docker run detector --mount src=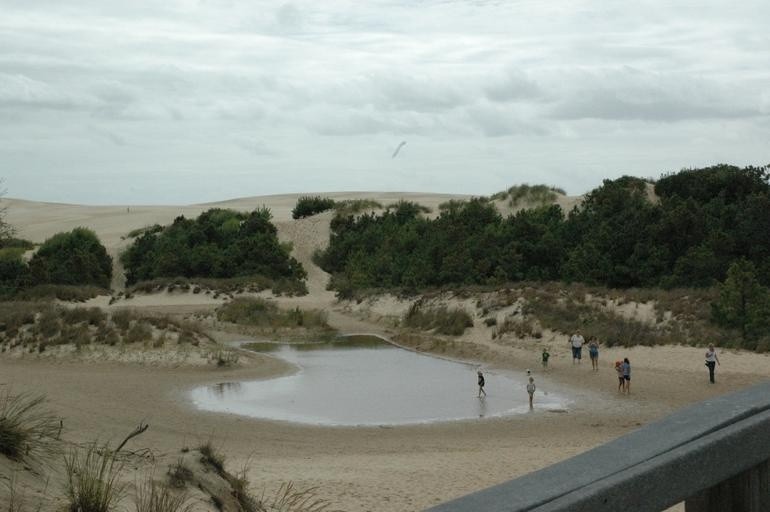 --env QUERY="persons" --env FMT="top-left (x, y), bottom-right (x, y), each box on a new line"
top-left (527, 377), bottom-right (536, 408)
top-left (477, 371), bottom-right (486, 398)
top-left (615, 358), bottom-right (631, 394)
top-left (571, 329), bottom-right (585, 365)
top-left (543, 348), bottom-right (550, 368)
top-left (587, 335), bottom-right (599, 370)
top-left (706, 343), bottom-right (720, 384)
